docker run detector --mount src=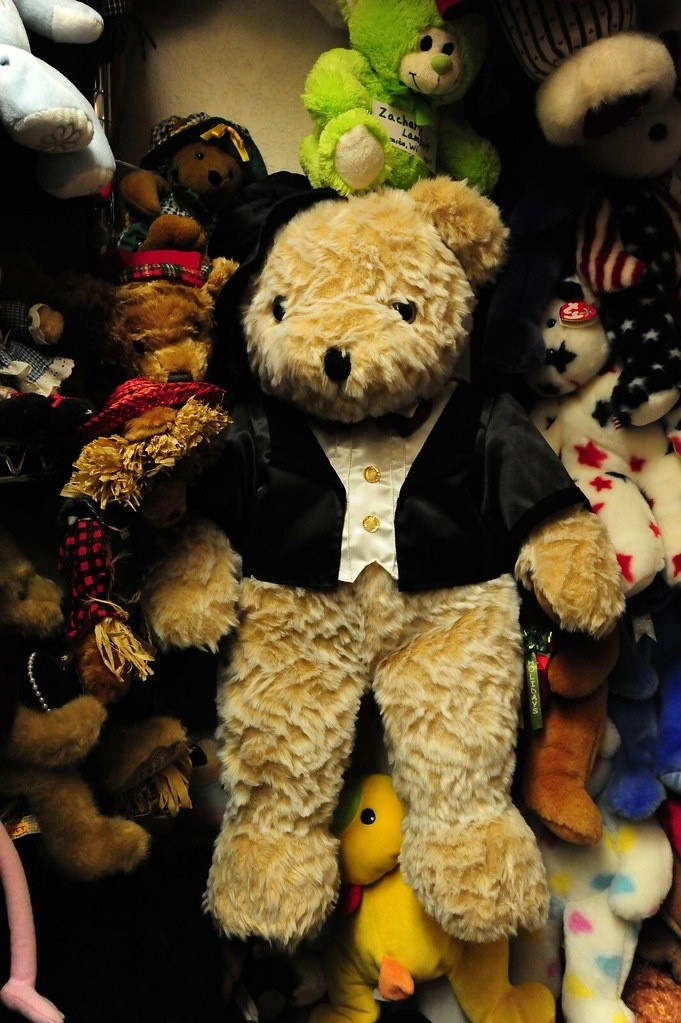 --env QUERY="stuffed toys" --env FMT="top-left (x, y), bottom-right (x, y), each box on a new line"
top-left (0, 0), bottom-right (681, 1023)
